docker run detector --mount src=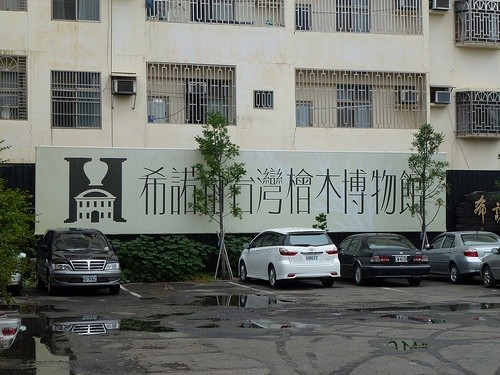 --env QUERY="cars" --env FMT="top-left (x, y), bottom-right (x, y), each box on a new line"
top-left (1, 313), bottom-right (26, 351)
top-left (240, 296), bottom-right (321, 330)
top-left (36, 228), bottom-right (120, 294)
top-left (419, 231), bottom-right (499, 282)
top-left (238, 227), bottom-right (340, 288)
top-left (480, 247), bottom-right (500, 287)
top-left (7, 251), bottom-right (27, 294)
top-left (337, 232), bottom-right (430, 286)
